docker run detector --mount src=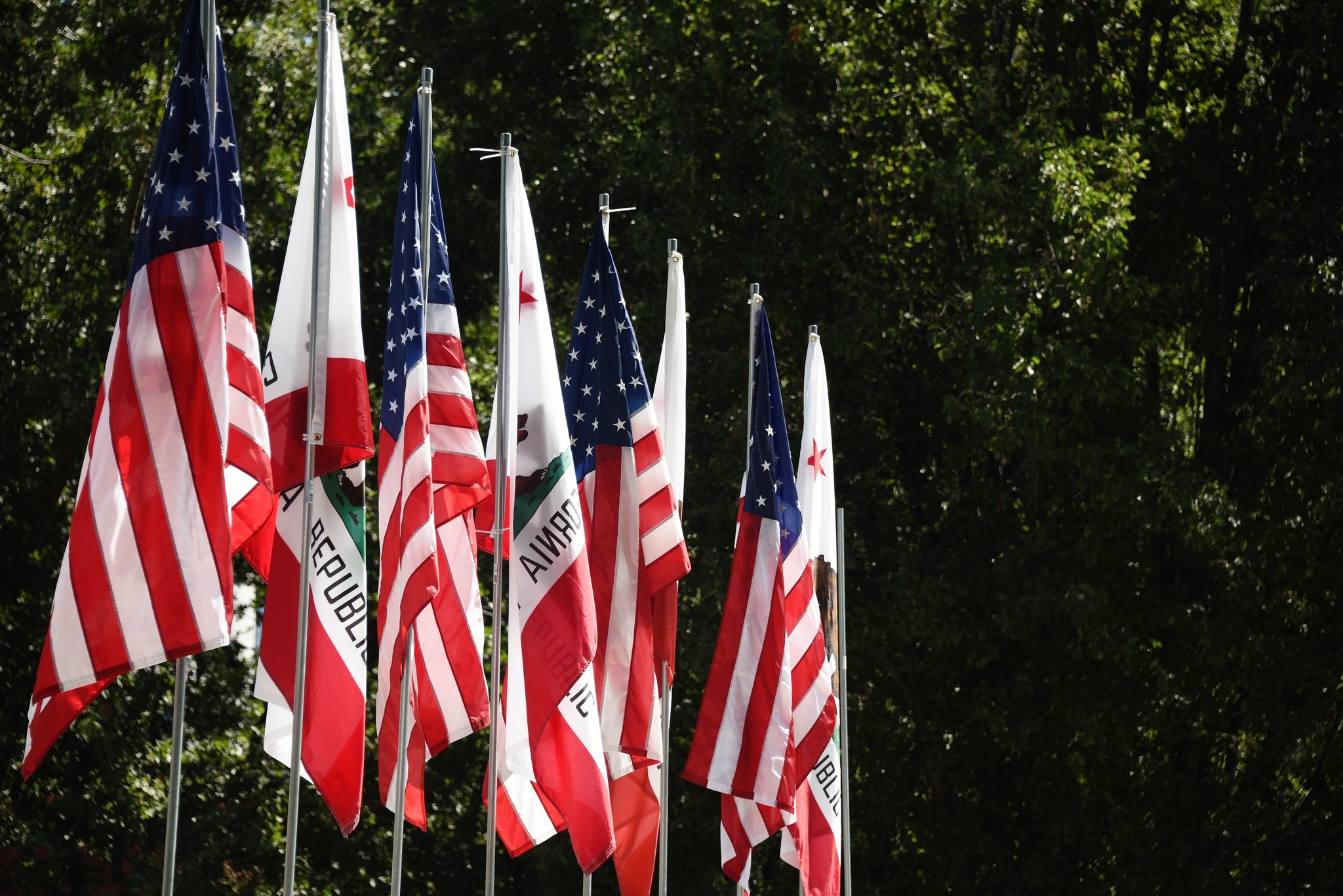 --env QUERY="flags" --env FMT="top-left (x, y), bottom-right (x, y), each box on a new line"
top-left (257, 11), bottom-right (373, 837)
top-left (681, 305), bottom-right (839, 896)
top-left (563, 209), bottom-right (690, 896)
top-left (479, 148), bottom-right (618, 880)
top-left (22, 0), bottom-right (273, 780)
top-left (650, 258), bottom-right (687, 803)
top-left (789, 330), bottom-right (844, 896)
top-left (377, 91), bottom-right (490, 829)
